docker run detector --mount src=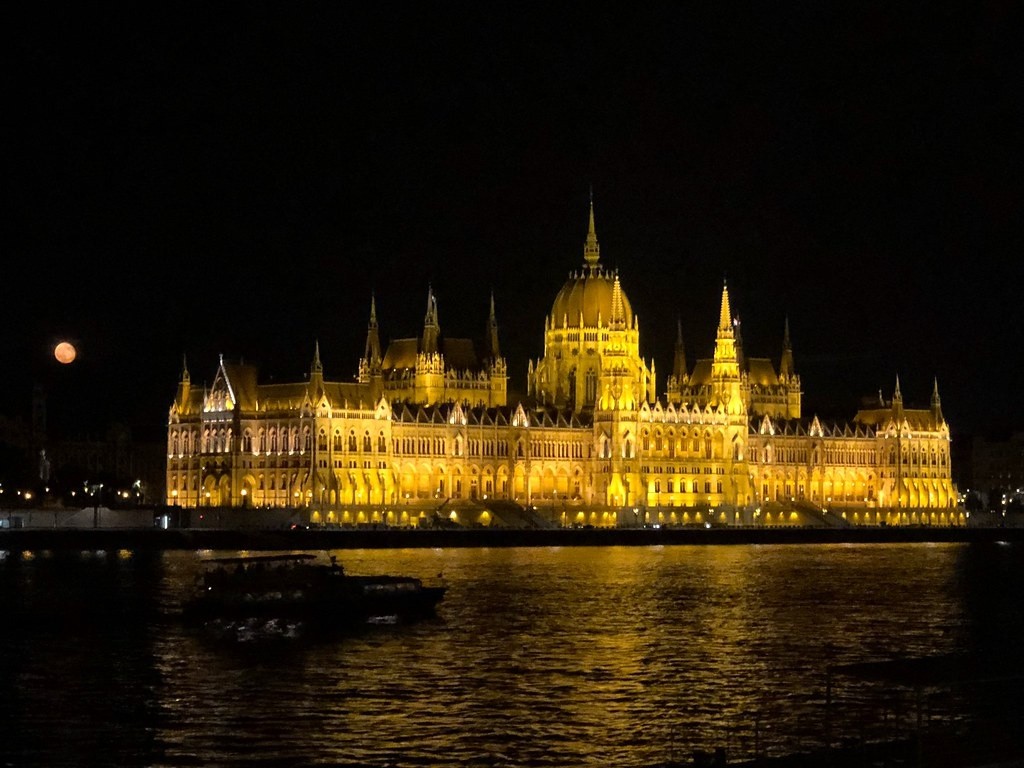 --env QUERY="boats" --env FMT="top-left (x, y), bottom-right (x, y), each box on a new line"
top-left (182, 554), bottom-right (447, 628)
